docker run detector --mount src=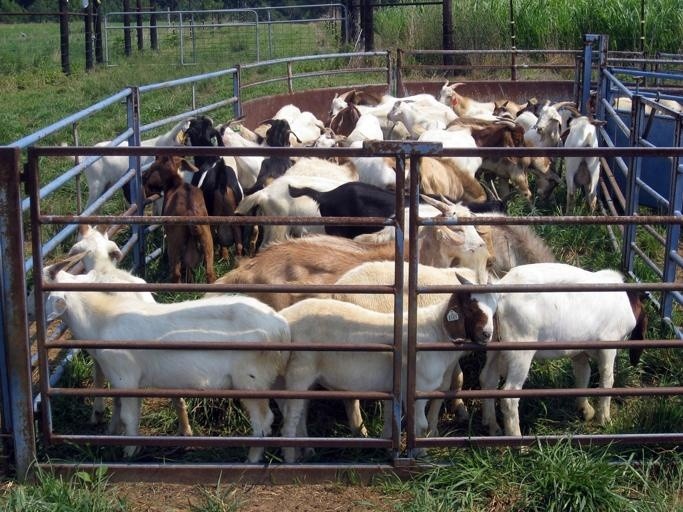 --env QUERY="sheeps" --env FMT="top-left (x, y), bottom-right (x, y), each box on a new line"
top-left (614, 93), bottom-right (681, 116)
top-left (19, 79), bottom-right (649, 462)
